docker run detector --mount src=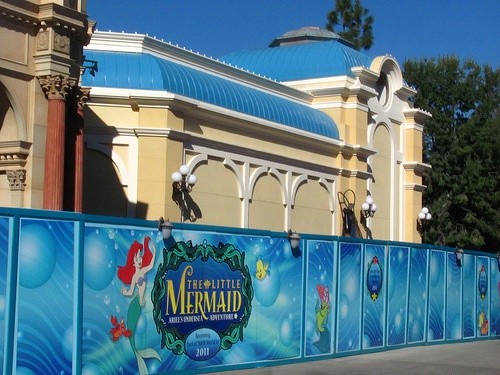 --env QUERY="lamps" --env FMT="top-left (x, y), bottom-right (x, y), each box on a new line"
top-left (416, 207), bottom-right (432, 232)
top-left (287, 229), bottom-right (300, 249)
top-left (361, 196), bottom-right (377, 218)
top-left (84, 59), bottom-right (99, 72)
top-left (454, 246), bottom-right (463, 260)
top-left (159, 216), bottom-right (174, 239)
top-left (171, 165), bottom-right (196, 194)
top-left (83, 65), bottom-right (96, 77)
